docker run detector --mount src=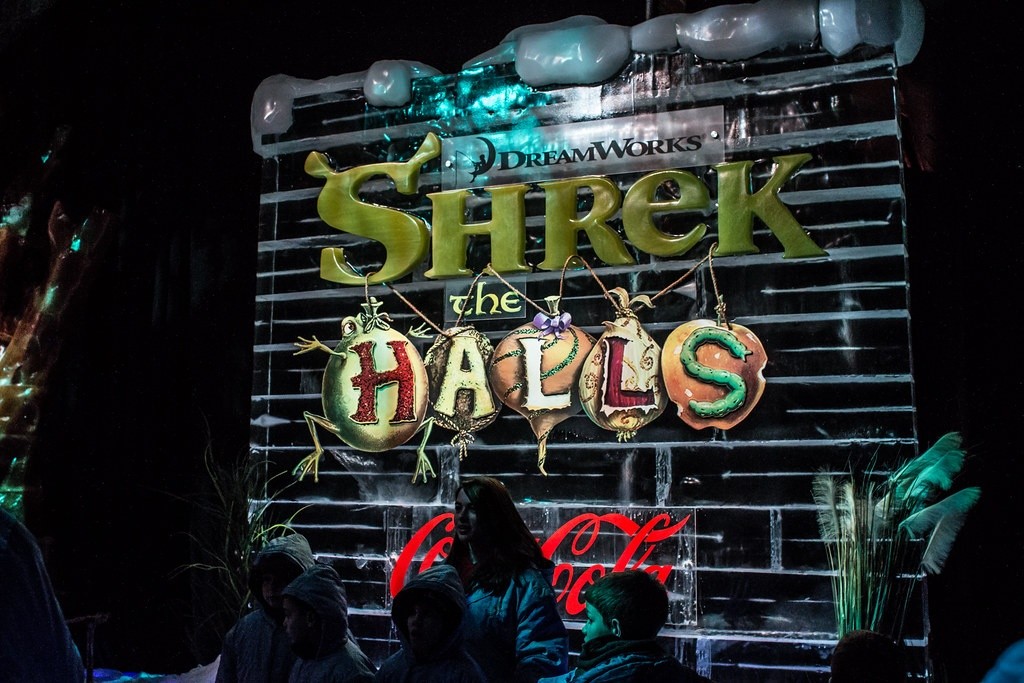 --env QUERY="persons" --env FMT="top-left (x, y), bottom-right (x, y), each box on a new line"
top-left (437, 478), bottom-right (569, 683)
top-left (213, 534), bottom-right (315, 683)
top-left (569, 572), bottom-right (718, 683)
top-left (276, 566), bottom-right (381, 681)
top-left (367, 563), bottom-right (488, 681)
